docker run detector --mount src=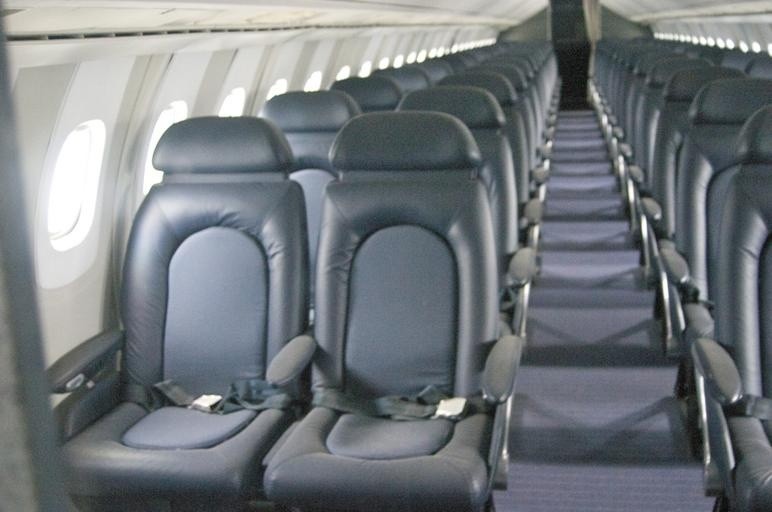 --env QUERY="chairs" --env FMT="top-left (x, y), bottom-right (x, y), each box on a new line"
top-left (51, 39), bottom-right (561, 509)
top-left (588, 34), bottom-right (770, 512)
